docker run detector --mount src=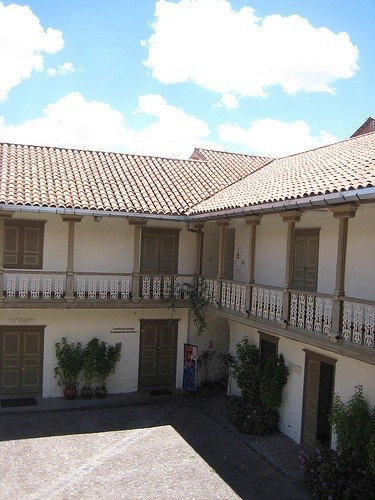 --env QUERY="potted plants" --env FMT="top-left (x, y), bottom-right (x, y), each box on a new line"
top-left (216, 334), bottom-right (289, 436)
top-left (78, 337), bottom-right (99, 400)
top-left (52, 337), bottom-right (81, 399)
top-left (93, 341), bottom-right (121, 398)
